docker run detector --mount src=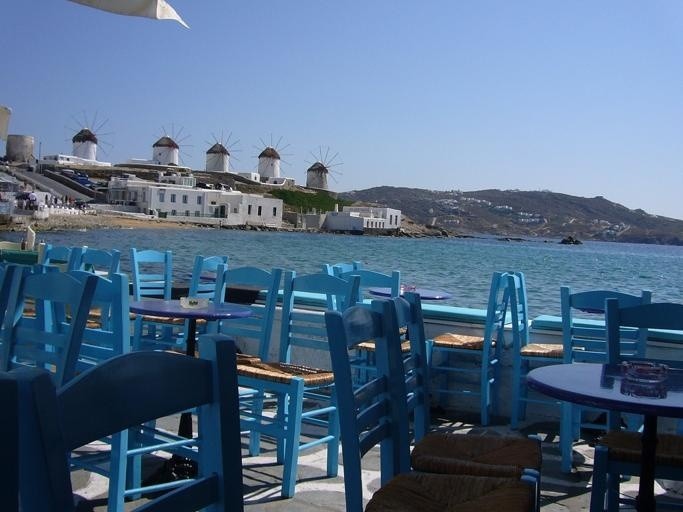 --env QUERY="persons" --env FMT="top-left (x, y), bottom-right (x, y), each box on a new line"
top-left (77, 172), bottom-right (89, 179)
top-left (28, 191), bottom-right (90, 210)
